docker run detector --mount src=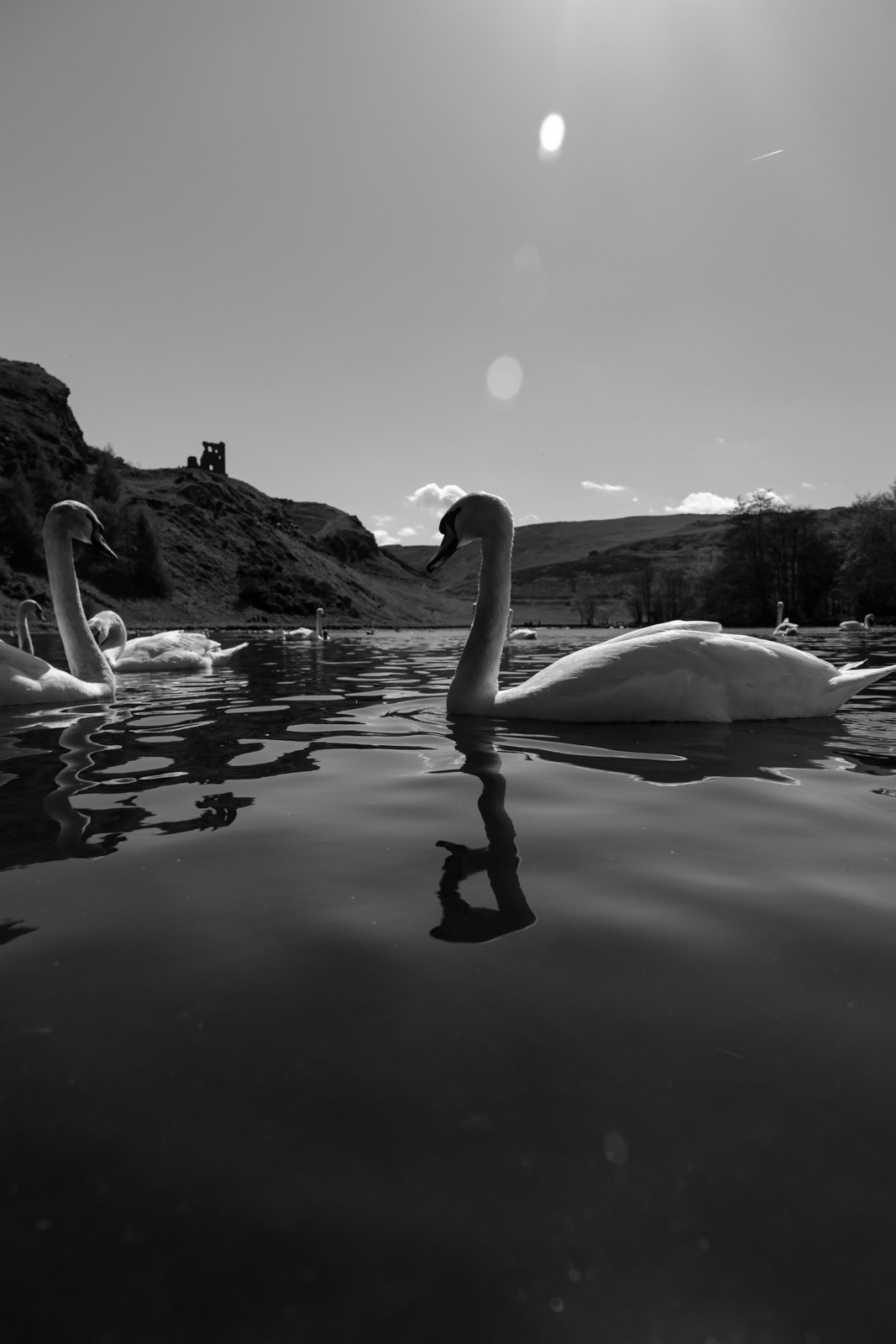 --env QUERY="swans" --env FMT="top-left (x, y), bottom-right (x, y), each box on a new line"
top-left (424, 487), bottom-right (896, 724)
top-left (0, 498), bottom-right (375, 707)
